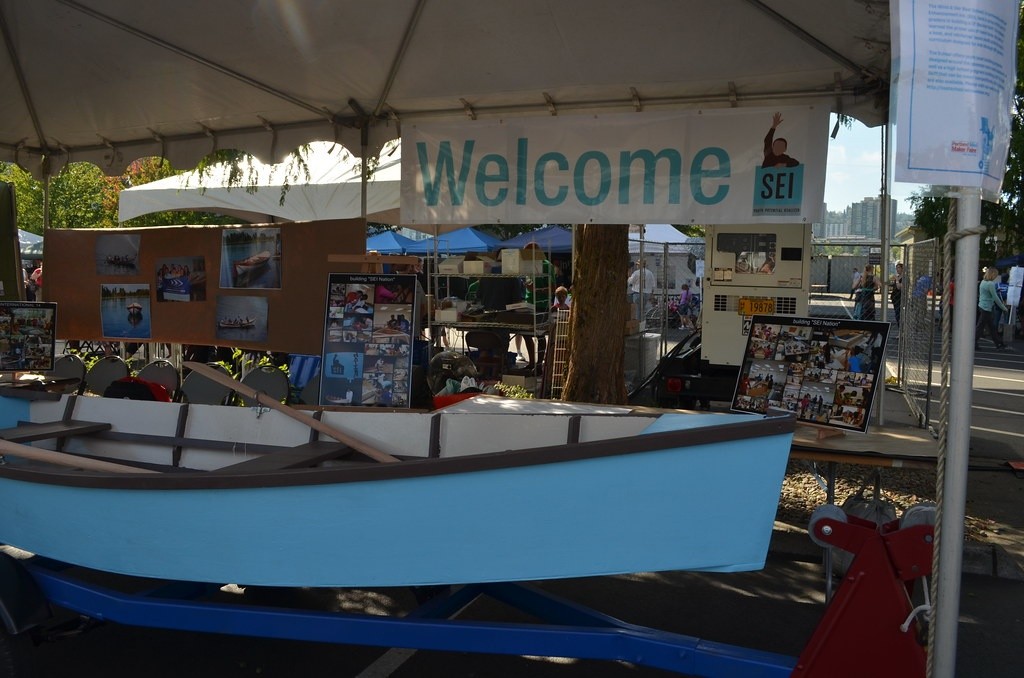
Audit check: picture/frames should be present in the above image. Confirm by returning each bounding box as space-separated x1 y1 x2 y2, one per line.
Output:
730 314 891 434
0 301 57 372
317 272 417 410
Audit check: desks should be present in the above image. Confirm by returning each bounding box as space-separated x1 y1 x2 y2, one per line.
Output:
625 329 649 379
431 395 946 608
0 371 81 392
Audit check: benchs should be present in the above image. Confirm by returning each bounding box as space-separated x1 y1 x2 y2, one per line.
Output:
0 419 111 444
209 441 354 475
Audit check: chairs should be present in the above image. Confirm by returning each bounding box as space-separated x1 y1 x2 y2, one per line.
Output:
135 359 181 403
177 363 233 406
299 374 319 405
233 366 292 406
43 355 87 395
77 355 130 400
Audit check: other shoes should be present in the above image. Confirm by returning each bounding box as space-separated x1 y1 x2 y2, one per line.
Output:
516 353 525 360
997 343 1008 349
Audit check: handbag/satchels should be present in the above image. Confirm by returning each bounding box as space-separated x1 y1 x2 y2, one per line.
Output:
26 279 39 294
677 303 686 315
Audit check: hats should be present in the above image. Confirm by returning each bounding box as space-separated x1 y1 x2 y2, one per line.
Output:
636 258 646 266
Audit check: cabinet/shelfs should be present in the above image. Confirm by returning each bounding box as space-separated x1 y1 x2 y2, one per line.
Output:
426 236 552 399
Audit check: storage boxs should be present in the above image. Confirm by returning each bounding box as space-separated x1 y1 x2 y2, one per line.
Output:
438 255 465 274
435 307 462 322
624 319 639 335
625 304 637 320
497 249 547 274
463 260 492 274
506 303 534 312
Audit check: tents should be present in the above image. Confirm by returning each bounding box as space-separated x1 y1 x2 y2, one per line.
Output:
363 222 689 334
118 139 403 227
17 228 48 259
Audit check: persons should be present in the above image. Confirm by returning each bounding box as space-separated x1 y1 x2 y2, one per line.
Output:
157 264 189 289
106 255 130 263
464 242 569 379
388 253 453 348
627 259 656 321
735 322 878 428
21 261 43 302
677 284 692 331
849 264 881 322
890 264 1010 352
0 309 54 369
330 279 412 405
